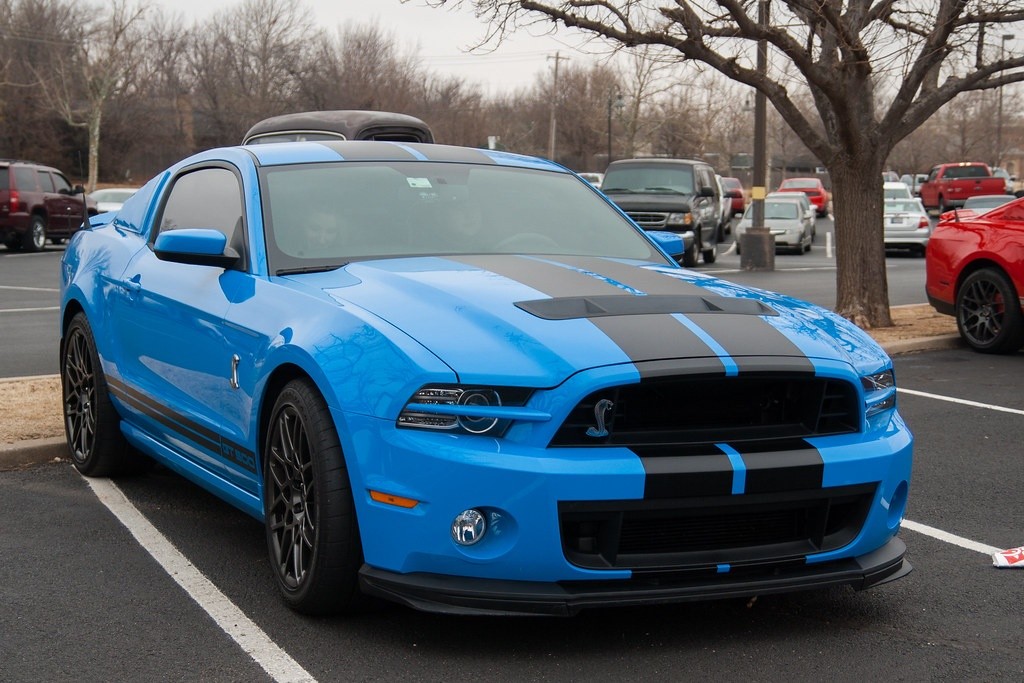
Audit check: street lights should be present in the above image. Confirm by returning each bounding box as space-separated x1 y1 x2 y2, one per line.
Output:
608 84 622 166
997 34 1015 168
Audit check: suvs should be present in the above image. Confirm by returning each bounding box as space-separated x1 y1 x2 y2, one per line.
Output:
0 160 98 252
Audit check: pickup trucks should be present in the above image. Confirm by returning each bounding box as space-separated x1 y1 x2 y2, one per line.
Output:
917 162 1008 218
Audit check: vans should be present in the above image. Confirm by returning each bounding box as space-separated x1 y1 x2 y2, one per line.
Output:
598 160 720 264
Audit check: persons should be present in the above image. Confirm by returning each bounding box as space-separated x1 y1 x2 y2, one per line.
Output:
451 193 483 237
298 199 347 250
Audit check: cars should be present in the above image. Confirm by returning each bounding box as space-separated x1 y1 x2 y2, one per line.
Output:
241 111 436 145
58 142 913 620
576 173 603 192
776 177 830 216
990 167 1017 194
722 178 745 215
766 192 817 235
87 188 141 213
715 174 735 241
884 197 932 252
882 172 923 195
883 182 912 212
733 200 813 253
925 196 1024 353
